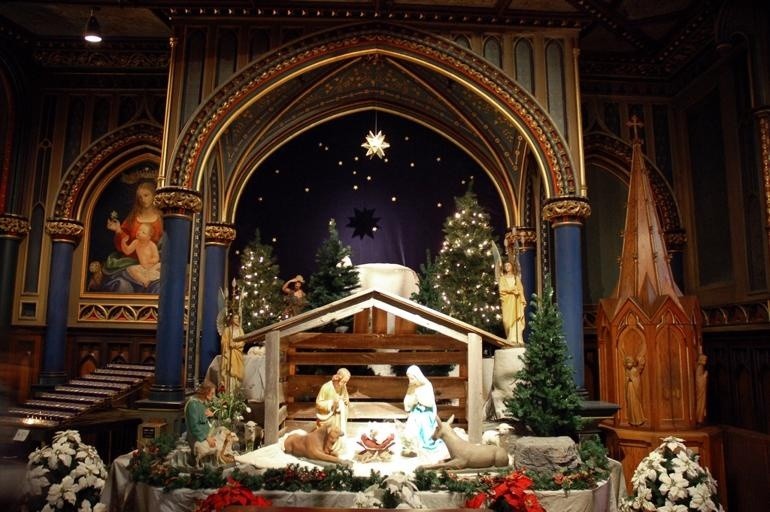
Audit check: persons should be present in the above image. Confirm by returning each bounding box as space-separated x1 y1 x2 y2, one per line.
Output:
184 378 216 449
315 368 351 455
121 223 159 288
404 365 442 450
624 357 648 426
103 183 163 291
695 354 708 423
283 275 307 298
498 262 527 345
220 313 245 391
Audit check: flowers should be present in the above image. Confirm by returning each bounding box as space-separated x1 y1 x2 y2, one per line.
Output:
21 429 108 511
125 432 611 511
619 436 726 512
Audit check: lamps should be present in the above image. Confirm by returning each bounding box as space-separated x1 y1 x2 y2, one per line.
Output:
82 1 102 44
360 110 391 161
